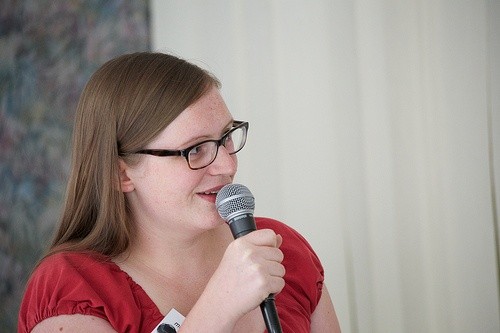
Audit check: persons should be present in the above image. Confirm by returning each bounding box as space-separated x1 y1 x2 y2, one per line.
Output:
15 51 341 333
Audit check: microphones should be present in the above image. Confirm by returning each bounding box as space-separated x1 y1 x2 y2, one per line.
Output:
216 183 286 333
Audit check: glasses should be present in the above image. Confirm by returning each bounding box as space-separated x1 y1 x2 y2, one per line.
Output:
123 119 250 171
